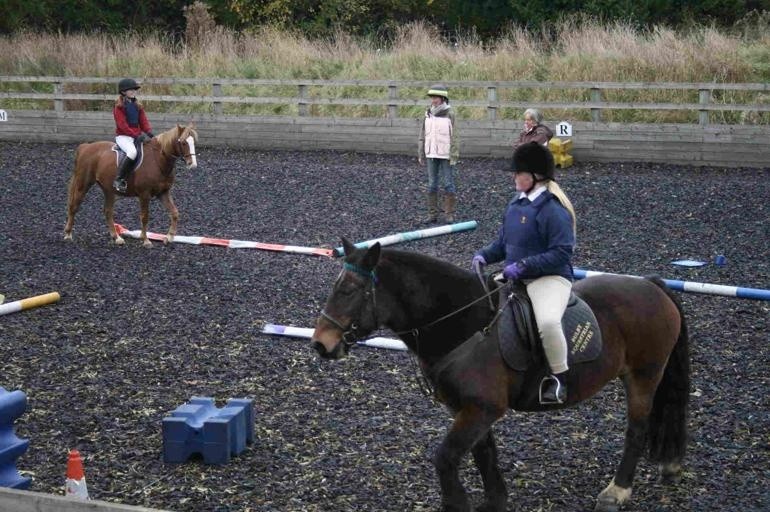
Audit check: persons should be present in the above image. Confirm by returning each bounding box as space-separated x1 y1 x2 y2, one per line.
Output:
473 140 576 402
521 107 554 146
418 83 460 222
113 79 155 193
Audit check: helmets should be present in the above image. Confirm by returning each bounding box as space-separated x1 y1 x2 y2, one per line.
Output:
119 79 141 93
426 84 448 100
502 140 555 181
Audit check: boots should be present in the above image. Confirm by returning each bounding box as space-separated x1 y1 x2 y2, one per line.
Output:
112 156 135 193
444 193 457 223
423 192 439 223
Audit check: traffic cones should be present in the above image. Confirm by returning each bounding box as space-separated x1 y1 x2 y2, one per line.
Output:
61 449 92 502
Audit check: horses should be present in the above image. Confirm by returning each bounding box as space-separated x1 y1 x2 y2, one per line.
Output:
62 120 199 250
310 235 690 512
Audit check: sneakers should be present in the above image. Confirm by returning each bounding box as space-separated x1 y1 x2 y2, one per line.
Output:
543 370 568 401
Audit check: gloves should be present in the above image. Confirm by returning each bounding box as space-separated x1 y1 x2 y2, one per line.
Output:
503 261 527 281
137 132 155 144
471 255 487 274
